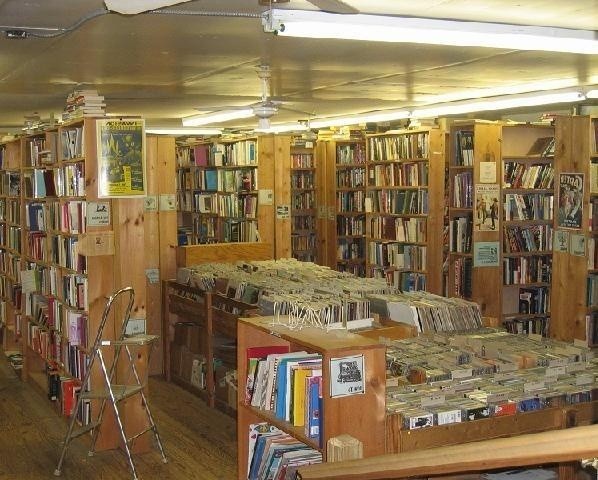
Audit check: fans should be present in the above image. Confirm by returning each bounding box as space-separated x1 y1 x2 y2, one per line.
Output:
182 64 330 117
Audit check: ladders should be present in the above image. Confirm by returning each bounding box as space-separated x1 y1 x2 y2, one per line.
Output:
53 286 168 480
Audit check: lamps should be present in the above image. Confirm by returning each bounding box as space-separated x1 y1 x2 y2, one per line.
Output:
260 1 598 56
182 106 254 127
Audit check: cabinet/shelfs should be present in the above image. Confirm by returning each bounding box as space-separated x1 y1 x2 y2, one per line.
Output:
2 115 151 455
160 242 497 421
234 315 598 480
146 112 596 376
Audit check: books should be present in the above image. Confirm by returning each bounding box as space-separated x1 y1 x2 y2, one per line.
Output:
501 132 598 346
368 291 597 427
289 134 317 263
447 130 471 299
174 139 257 244
65 89 106 118
0 127 91 427
174 255 400 323
245 345 322 480
179 350 238 408
366 136 426 290
336 144 366 277
